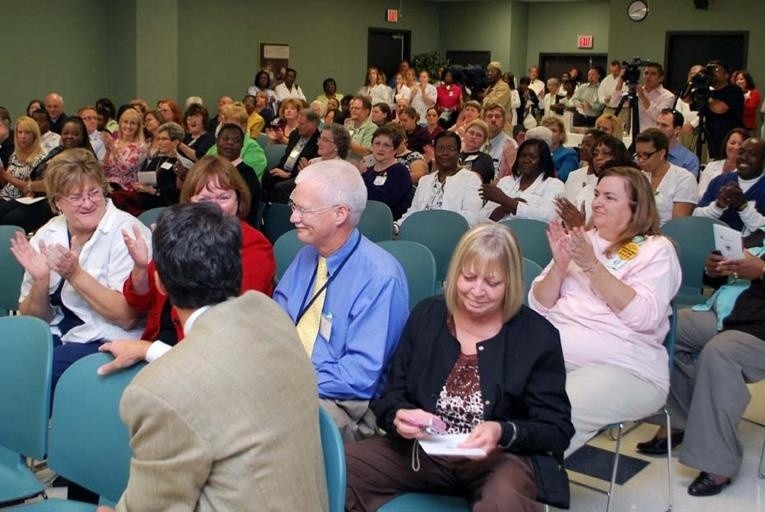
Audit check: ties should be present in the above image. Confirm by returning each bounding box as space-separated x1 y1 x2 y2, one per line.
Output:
296 254 328 360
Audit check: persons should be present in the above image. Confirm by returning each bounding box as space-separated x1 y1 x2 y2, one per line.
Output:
1 55 765 511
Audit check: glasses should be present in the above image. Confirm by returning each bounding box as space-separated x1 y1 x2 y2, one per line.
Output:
60 187 104 207
632 148 660 160
287 200 337 218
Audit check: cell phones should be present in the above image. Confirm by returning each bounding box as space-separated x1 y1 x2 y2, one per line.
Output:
711 250 723 261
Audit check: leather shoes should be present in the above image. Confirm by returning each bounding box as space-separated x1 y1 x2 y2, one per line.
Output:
637 431 684 453
688 471 731 496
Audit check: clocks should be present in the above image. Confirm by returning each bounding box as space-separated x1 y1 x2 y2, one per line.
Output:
627 0 650 22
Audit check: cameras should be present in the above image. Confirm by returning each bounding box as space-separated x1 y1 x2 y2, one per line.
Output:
406 414 447 435
621 56 650 84
679 63 720 102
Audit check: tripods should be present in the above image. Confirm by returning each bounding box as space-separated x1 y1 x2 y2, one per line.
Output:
689 102 721 183
613 84 640 152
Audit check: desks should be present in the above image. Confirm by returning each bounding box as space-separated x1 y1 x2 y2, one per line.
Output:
554 130 633 153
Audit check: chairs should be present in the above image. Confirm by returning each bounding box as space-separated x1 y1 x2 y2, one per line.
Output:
0 314 59 508
0 223 30 317
11 340 158 512
131 206 170 233
251 127 364 179
